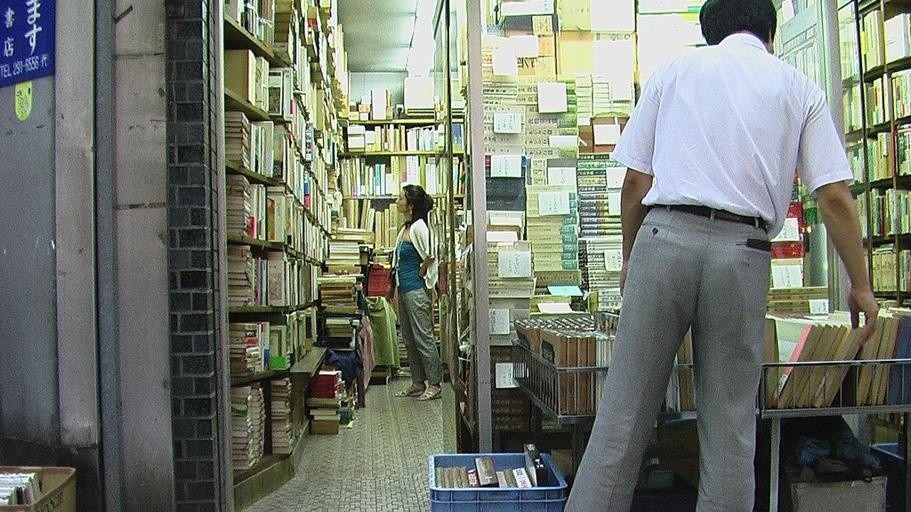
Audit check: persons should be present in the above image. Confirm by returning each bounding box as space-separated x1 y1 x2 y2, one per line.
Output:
385 185 443 401
561 1 880 510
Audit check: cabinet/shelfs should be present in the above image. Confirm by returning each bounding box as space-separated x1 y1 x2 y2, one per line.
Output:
224 1 911 512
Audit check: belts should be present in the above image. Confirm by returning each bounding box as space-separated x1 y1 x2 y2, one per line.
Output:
651 202 767 229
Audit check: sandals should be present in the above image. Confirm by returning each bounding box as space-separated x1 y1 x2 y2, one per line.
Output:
393 382 427 396
416 383 443 402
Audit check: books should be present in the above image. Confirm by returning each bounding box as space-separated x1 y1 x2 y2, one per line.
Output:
224 1 911 491
0 468 44 505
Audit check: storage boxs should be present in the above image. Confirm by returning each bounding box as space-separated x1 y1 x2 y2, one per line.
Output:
1 466 76 512
